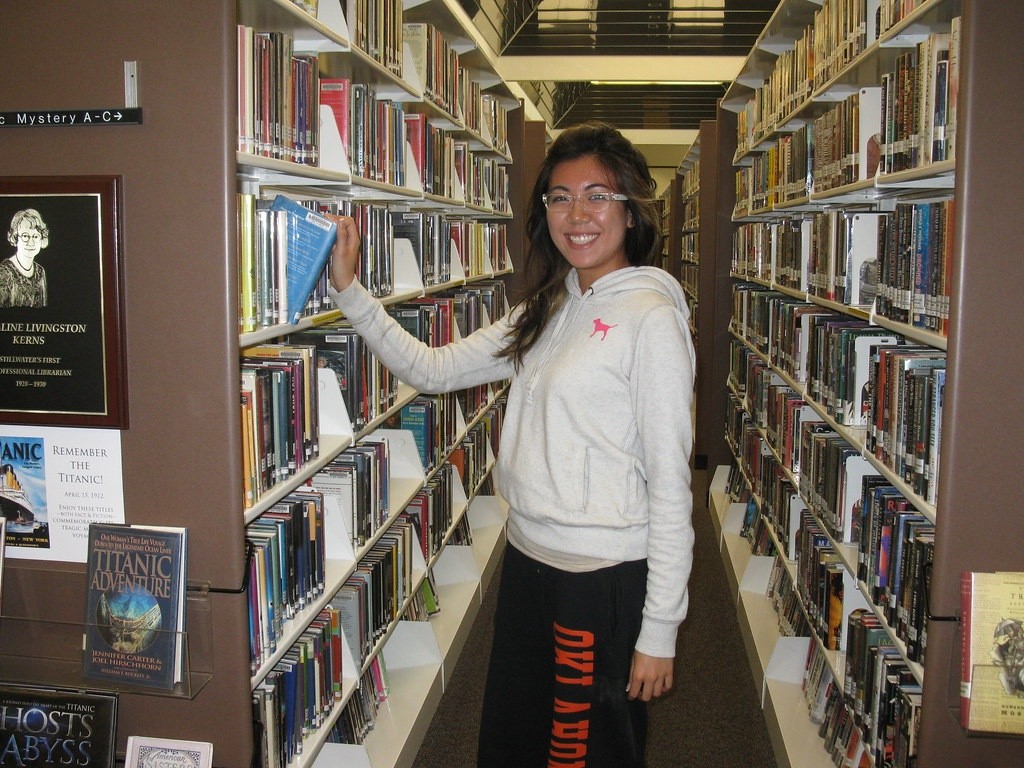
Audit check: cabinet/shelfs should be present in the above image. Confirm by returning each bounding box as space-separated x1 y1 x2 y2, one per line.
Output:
0 2 556 767
649 1 1024 768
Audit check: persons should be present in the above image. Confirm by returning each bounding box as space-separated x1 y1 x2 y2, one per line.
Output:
320 123 697 768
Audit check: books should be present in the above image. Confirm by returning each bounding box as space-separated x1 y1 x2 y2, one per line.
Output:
236 0 1023 767
83 522 189 690
0 681 120 768
125 736 214 768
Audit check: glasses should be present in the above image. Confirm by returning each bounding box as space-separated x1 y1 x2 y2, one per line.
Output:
541 192 629 213
